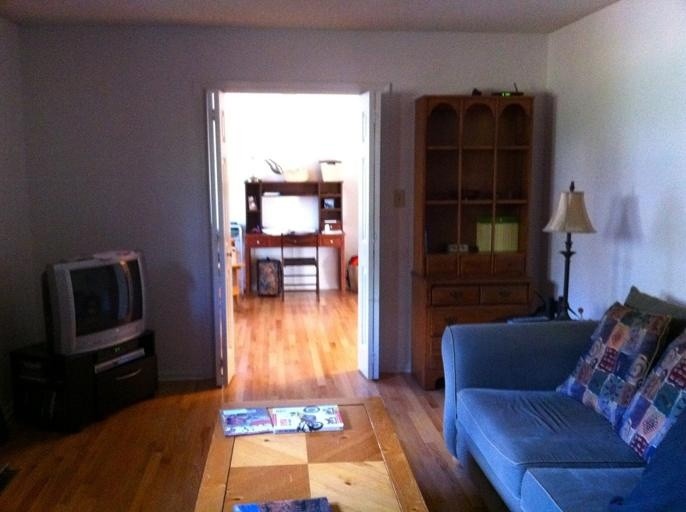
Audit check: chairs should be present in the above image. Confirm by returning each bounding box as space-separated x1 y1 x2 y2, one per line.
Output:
279 233 319 301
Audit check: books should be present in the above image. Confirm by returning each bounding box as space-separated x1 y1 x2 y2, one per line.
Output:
219 406 274 437
266 402 345 433
234 497 330 511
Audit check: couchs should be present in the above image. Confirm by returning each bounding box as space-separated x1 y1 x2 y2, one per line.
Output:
440 287 686 511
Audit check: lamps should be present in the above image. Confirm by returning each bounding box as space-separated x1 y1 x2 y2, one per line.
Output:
541 180 598 321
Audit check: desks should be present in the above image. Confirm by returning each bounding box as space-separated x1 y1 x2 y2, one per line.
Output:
244 231 346 297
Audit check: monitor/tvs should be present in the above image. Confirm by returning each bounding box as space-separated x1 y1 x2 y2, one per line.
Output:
41 251 145 357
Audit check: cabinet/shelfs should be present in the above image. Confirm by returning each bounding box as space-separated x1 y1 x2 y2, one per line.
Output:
7 327 158 432
247 179 343 233
413 93 536 278
410 271 533 390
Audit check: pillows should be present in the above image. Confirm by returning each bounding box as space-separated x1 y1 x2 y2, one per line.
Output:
555 300 686 465
